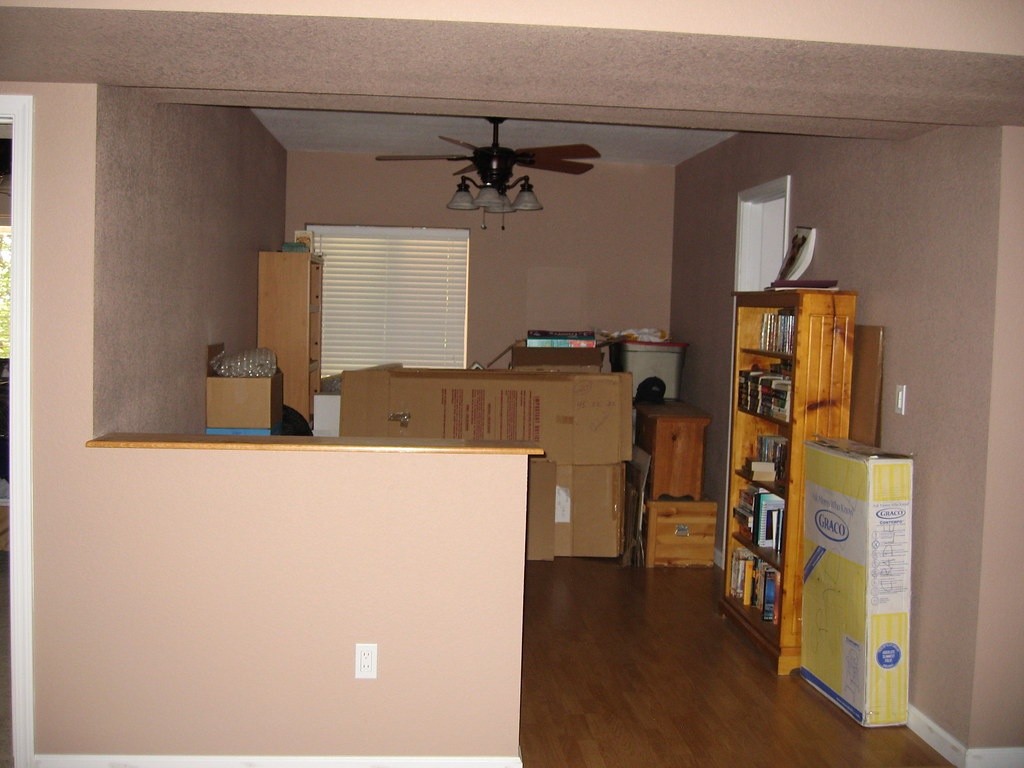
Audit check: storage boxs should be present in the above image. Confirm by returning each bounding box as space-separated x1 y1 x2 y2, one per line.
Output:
803 433 914 728
206 343 284 436
312 331 721 568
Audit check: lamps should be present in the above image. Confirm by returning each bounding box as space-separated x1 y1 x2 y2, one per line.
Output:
447 178 543 231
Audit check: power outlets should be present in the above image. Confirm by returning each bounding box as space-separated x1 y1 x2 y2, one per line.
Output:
353 644 378 678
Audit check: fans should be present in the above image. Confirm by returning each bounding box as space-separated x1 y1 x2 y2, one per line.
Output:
375 117 601 230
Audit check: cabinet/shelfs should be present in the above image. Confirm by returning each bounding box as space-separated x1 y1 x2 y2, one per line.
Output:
718 286 860 677
635 403 714 501
256 251 323 431
